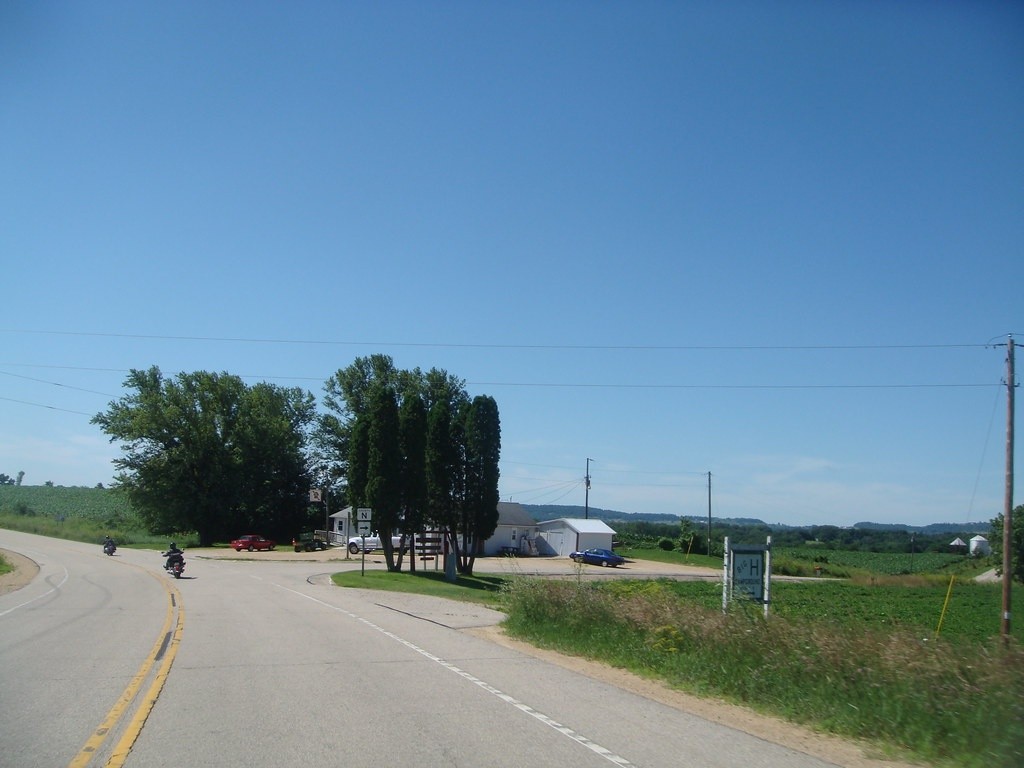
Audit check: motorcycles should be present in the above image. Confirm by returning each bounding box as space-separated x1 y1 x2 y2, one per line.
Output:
103 539 116 556
161 548 186 579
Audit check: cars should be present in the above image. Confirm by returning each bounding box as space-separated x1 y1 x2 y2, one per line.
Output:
231 535 275 552
569 548 625 567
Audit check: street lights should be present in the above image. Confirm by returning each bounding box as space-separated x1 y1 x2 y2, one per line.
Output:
586 458 594 518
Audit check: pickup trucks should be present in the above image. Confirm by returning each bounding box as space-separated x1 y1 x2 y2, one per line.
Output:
349 530 411 555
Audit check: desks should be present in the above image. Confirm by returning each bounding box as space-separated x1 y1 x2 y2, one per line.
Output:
501 547 520 557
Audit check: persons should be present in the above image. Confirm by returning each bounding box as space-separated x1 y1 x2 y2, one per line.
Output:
163 542 185 569
102 535 114 550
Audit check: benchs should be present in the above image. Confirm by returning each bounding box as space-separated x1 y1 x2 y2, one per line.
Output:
497 551 518 552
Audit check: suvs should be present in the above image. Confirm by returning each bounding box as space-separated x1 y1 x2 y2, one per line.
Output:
292 532 328 552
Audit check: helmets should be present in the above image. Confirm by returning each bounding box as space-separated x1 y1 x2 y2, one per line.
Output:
105 535 109 539
170 542 176 549
